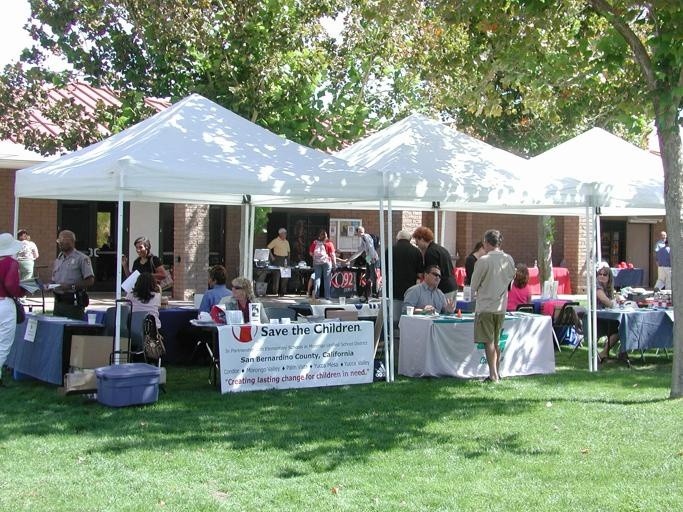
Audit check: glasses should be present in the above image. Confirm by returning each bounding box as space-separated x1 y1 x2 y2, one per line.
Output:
232 285 242 289
431 271 441 277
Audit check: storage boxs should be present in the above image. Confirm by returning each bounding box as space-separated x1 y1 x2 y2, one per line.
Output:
90 362 162 409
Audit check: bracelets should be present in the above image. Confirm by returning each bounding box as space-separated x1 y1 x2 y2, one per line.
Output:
71 284 75 291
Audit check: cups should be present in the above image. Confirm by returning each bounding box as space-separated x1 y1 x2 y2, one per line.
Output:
87 313 95 324
269 317 278 324
339 296 344 305
161 294 168 309
197 311 211 320
406 305 413 317
280 317 290 324
542 280 559 299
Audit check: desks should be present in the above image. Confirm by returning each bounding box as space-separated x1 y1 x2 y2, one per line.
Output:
5 261 675 396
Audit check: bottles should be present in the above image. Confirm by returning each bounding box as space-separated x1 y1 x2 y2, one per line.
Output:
283 258 287 267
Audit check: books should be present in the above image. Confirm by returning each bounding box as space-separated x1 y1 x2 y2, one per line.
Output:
20 277 43 294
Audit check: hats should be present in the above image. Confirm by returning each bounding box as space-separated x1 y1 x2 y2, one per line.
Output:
0 233 22 257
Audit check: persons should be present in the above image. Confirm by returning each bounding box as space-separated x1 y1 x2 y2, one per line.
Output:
44 229 95 319
380 230 423 337
267 228 291 296
130 237 168 280
401 264 456 314
123 271 162 330
582 267 632 367
466 241 486 282
218 276 269 324
654 240 671 290
413 228 457 302
305 231 348 299
653 230 667 253
470 230 515 382
507 263 530 310
0 232 28 392
346 227 379 295
196 265 232 318
17 229 38 281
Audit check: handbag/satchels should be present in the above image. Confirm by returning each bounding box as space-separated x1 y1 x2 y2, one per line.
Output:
56 289 89 307
14 299 25 324
363 233 380 250
143 315 166 359
150 256 173 290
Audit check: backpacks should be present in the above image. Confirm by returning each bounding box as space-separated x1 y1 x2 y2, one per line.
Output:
313 240 328 265
